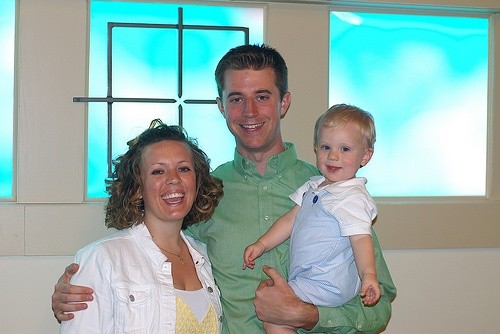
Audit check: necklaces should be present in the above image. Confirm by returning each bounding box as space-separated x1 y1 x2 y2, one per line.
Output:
156 241 185 265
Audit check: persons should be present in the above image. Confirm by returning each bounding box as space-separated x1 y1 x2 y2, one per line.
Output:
51 44 396 334
242 103 382 334
59 118 224 334
